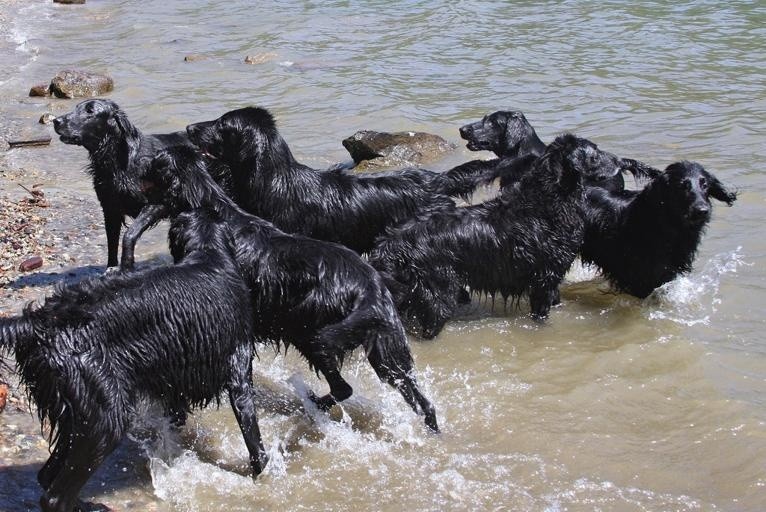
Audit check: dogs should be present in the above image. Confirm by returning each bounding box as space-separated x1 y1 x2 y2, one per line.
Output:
0 207 269 512
53 99 235 271
135 142 439 434
580 160 742 299
361 133 626 340
459 111 662 192
186 107 501 305
